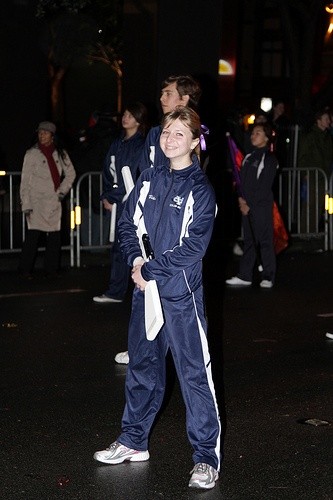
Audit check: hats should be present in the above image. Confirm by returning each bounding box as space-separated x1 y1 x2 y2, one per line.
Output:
36 122 56 132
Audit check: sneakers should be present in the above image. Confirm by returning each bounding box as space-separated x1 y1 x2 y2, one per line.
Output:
94 441 150 464
115 351 130 364
260 280 273 288
189 463 219 489
93 294 122 303
326 329 333 338
226 277 251 286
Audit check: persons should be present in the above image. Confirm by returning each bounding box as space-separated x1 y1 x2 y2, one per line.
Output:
92 104 222 489
115 75 208 365
225 123 278 288
234 98 333 254
93 105 145 303
17 121 76 282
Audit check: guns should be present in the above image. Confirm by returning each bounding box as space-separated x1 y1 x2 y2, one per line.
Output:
121 166 164 341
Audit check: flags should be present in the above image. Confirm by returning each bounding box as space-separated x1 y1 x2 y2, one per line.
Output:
226 132 288 254
